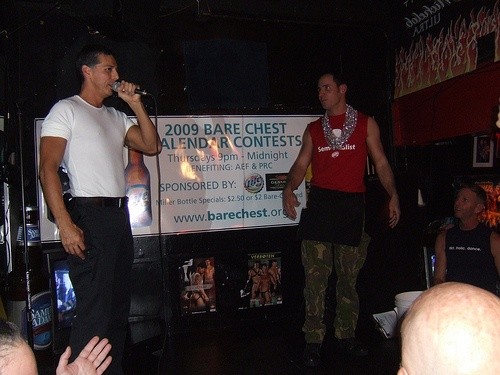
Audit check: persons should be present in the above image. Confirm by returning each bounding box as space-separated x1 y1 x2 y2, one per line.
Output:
245 259 282 305
182 259 215 309
283 71 400 367
397 281 500 375
0 320 113 375
434 185 500 296
39 48 161 375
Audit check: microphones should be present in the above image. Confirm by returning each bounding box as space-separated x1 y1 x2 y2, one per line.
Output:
112 82 153 97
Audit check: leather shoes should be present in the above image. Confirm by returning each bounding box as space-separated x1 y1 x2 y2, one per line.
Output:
303 343 320 367
336 337 368 355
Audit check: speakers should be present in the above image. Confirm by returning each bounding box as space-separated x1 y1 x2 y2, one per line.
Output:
130 253 163 343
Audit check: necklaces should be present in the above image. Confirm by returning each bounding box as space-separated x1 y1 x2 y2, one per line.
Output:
323 103 357 149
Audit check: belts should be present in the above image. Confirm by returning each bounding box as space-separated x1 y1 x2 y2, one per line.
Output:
68 197 127 208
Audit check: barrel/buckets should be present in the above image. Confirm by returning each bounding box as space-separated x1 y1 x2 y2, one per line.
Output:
395 291 423 329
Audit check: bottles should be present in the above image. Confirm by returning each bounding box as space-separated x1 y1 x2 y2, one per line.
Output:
13 202 56 366
124 145 153 227
